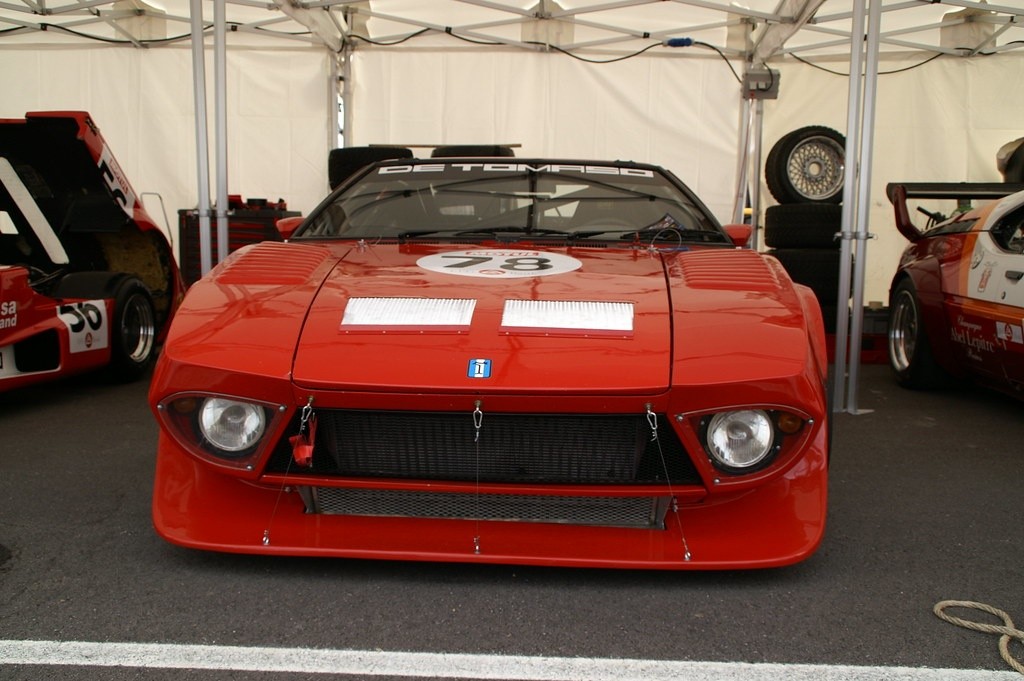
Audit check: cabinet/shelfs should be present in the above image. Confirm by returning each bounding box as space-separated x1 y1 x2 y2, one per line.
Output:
177 210 301 288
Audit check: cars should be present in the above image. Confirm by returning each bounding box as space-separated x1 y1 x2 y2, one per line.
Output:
886 136 1024 390
0 109 186 405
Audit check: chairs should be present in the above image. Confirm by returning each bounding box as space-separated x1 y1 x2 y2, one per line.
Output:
565 181 653 232
347 180 439 229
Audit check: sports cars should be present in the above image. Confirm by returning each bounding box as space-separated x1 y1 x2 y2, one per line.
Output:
150 142 831 574
